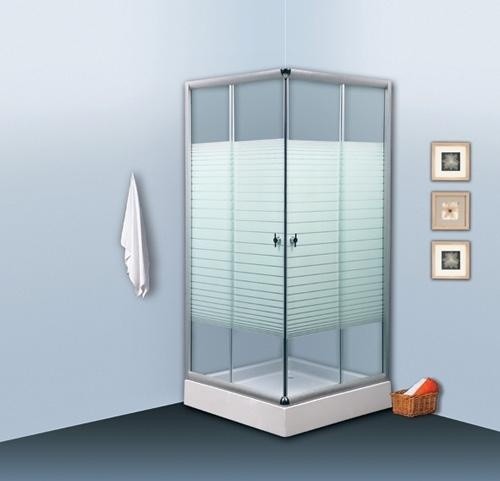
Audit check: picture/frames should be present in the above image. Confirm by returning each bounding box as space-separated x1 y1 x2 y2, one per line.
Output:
431 141 473 281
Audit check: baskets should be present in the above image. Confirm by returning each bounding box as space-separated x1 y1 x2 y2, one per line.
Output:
388 386 439 418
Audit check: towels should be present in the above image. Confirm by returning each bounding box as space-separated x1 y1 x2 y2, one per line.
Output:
121 170 152 299
